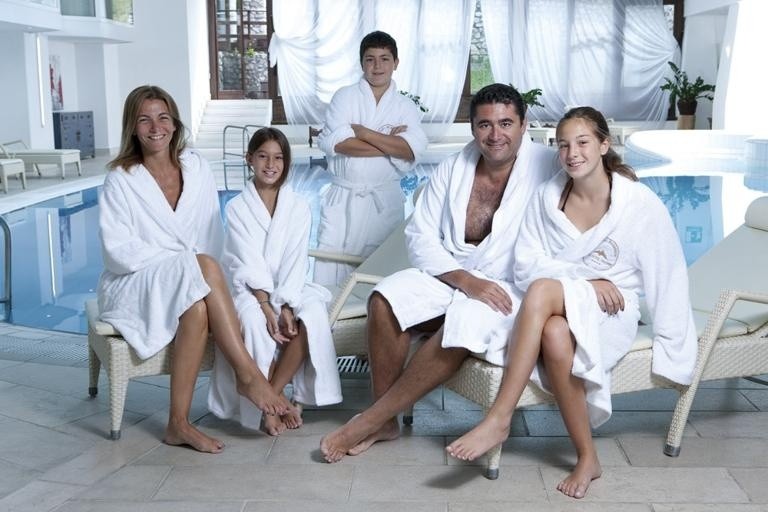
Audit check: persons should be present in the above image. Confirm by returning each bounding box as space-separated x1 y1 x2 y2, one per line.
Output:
445 106 700 500
320 83 567 462
208 126 343 436
315 31 427 285
97 82 289 453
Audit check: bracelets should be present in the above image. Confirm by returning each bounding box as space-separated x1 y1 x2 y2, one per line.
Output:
259 301 270 304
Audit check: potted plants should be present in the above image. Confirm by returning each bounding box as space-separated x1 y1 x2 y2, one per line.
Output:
659 60 715 115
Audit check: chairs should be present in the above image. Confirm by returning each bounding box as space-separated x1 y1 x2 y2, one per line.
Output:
1 139 82 195
86 182 427 438
398 193 768 479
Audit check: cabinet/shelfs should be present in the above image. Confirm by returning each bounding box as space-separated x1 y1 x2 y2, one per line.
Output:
52 111 95 160
